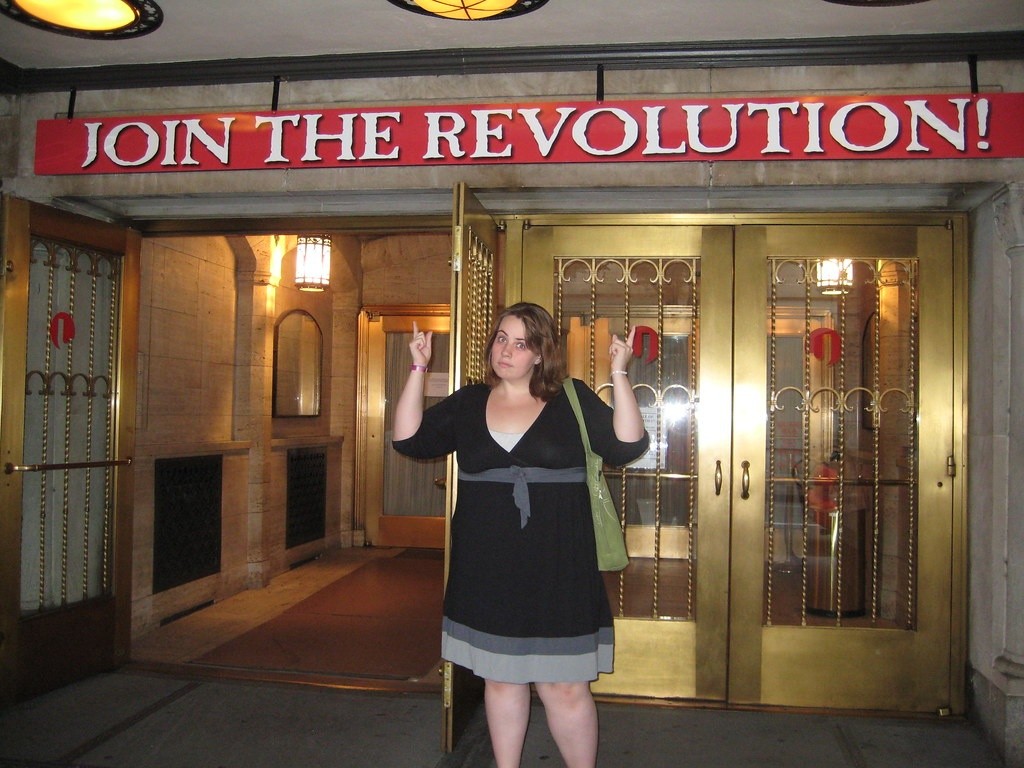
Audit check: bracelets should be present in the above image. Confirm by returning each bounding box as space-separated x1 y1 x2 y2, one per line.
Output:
408 363 427 375
609 370 629 377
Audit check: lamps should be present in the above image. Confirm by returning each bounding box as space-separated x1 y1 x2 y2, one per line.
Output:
387 0 550 21
0 0 164 40
816 257 855 297
294 234 333 293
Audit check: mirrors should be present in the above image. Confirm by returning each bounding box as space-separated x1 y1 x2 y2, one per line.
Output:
271 306 324 418
861 311 876 432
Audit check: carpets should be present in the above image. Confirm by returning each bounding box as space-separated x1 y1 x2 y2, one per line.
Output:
189 548 445 679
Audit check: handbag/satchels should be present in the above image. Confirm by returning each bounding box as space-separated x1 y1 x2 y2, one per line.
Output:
560 377 630 572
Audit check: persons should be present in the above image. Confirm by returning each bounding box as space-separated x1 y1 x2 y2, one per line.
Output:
393 303 648 768
